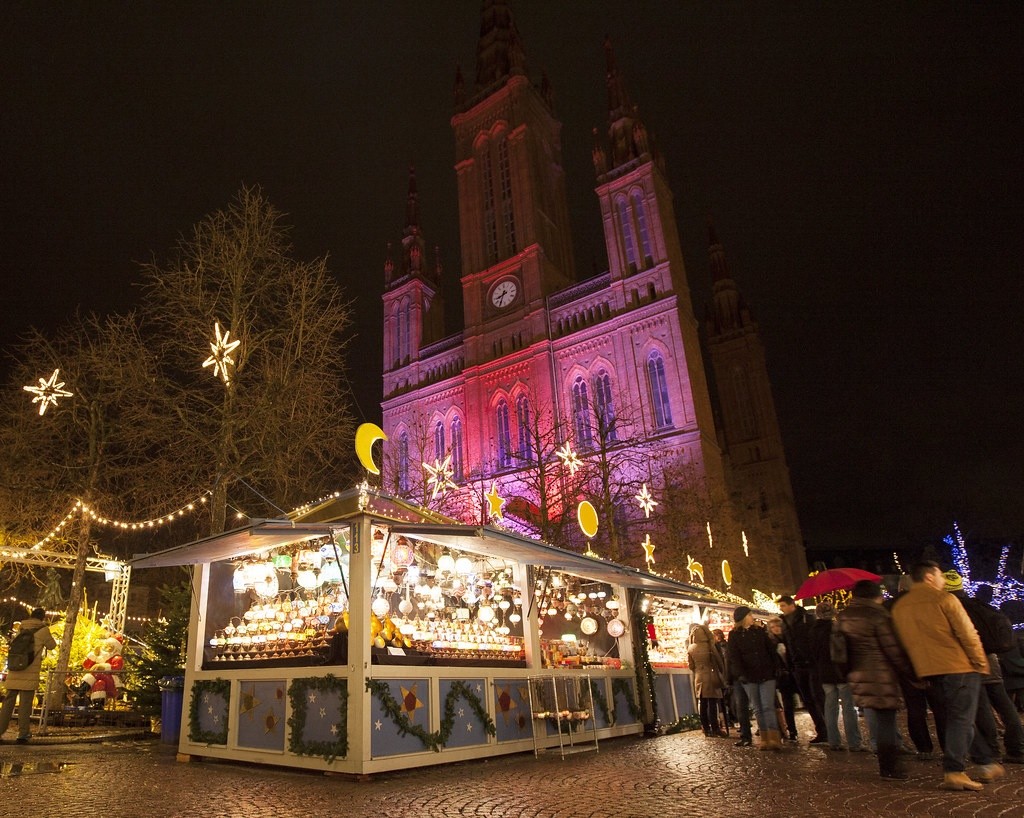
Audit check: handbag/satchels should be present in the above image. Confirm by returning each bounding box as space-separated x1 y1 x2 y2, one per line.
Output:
710 665 722 689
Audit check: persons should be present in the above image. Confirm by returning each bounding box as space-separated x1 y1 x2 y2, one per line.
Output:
810 603 867 751
768 616 801 742
974 585 1024 714
884 574 943 760
688 625 727 739
942 570 1024 764
727 607 781 751
712 630 741 729
70 636 124 710
830 580 917 781
892 560 1005 787
0 608 55 737
777 596 827 744
685 623 705 733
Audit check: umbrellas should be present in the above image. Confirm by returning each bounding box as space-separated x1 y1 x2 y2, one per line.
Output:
794 562 883 606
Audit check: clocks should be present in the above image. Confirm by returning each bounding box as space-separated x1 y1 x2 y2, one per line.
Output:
491 281 516 308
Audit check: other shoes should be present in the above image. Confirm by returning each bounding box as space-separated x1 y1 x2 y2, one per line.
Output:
711 725 726 738
827 745 848 751
701 726 712 738
881 767 909 781
16 733 32 740
1001 752 1024 764
977 762 1006 783
735 737 752 747
727 721 735 728
848 744 869 752
915 749 933 761
788 731 798 742
943 770 984 791
986 748 1003 763
809 735 829 744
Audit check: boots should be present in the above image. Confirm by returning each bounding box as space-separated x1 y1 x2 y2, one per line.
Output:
776 708 790 745
758 727 782 751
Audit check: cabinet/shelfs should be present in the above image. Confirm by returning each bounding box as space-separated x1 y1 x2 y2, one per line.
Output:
528 672 599 760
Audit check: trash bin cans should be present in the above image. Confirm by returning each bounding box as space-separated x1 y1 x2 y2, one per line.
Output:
159 686 184 745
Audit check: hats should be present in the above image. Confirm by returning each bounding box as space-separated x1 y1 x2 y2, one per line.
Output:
852 580 881 599
734 606 752 623
942 569 963 592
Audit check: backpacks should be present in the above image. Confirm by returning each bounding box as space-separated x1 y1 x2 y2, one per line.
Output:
7 625 47 672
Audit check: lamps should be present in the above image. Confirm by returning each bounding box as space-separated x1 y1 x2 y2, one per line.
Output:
210 534 619 661
